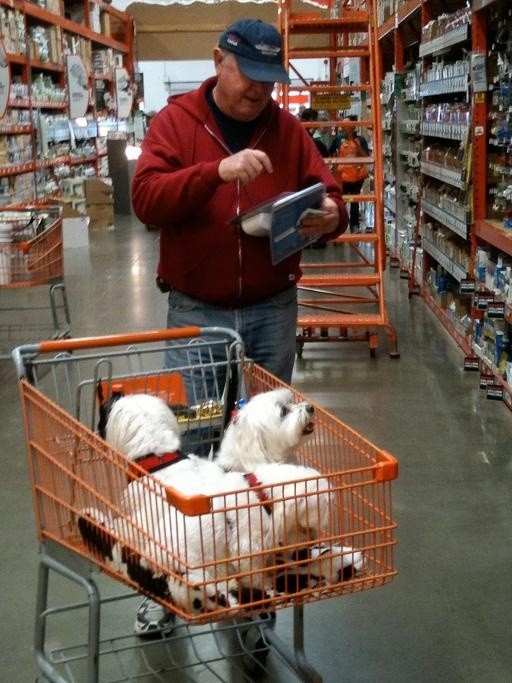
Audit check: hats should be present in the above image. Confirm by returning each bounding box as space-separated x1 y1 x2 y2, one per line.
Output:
219 19 292 85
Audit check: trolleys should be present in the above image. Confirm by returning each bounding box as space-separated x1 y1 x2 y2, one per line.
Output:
0 201 78 387
2 321 409 682
275 0 405 366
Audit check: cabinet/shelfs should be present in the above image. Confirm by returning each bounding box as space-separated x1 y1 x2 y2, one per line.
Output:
361 0 511 412
0 1 135 219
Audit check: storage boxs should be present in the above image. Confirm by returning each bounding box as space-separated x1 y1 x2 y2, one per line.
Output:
87 204 115 230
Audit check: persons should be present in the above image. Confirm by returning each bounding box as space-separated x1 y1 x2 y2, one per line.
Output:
293 102 371 250
130 19 350 671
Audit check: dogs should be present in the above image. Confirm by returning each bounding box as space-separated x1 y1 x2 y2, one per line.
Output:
75 386 370 625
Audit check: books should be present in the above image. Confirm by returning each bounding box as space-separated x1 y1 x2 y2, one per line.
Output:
268 182 325 267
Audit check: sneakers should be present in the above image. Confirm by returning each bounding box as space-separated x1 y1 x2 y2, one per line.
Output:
133 596 173 638
